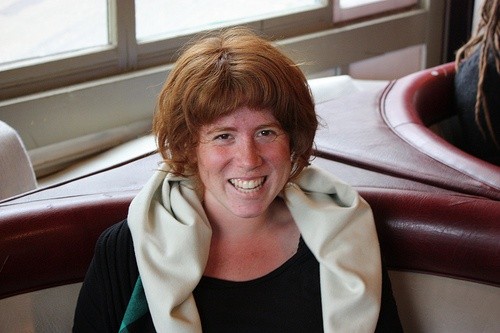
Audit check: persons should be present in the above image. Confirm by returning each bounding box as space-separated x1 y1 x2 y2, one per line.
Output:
446 0 500 167
70 24 407 333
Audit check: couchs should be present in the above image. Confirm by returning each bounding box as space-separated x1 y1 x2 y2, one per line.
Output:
0 53 500 332
